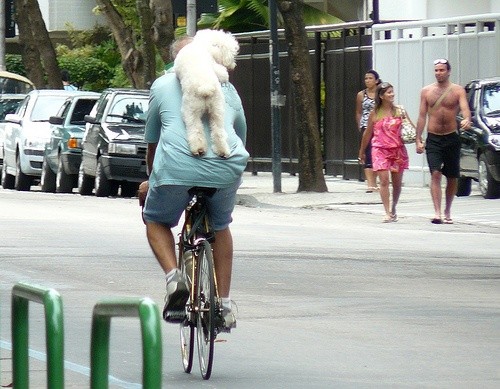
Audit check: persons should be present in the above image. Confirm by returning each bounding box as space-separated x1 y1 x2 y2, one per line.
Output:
416 59 471 224
138 36 249 329
359 82 423 223
356 70 382 193
60 70 78 91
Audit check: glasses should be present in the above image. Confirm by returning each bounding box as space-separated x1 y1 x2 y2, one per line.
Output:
433 58 449 65
381 82 392 88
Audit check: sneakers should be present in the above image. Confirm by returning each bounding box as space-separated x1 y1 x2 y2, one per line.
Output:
163 272 190 324
216 299 238 333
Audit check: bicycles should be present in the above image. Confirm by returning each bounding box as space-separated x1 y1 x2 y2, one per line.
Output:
143 185 223 379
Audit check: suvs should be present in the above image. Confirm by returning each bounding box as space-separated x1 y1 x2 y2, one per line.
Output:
453 77 500 199
78 88 153 197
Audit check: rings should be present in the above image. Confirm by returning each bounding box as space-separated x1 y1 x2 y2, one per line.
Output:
358 158 361 161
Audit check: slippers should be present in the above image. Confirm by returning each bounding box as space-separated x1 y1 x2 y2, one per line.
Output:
367 186 373 193
443 218 453 224
391 212 398 222
431 217 442 224
382 216 390 223
373 186 380 192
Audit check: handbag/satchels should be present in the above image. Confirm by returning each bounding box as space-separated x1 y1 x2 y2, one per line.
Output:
401 117 417 144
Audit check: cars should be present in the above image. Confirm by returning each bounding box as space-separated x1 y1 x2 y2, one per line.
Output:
0 94 30 185
2 90 97 191
41 94 101 193
